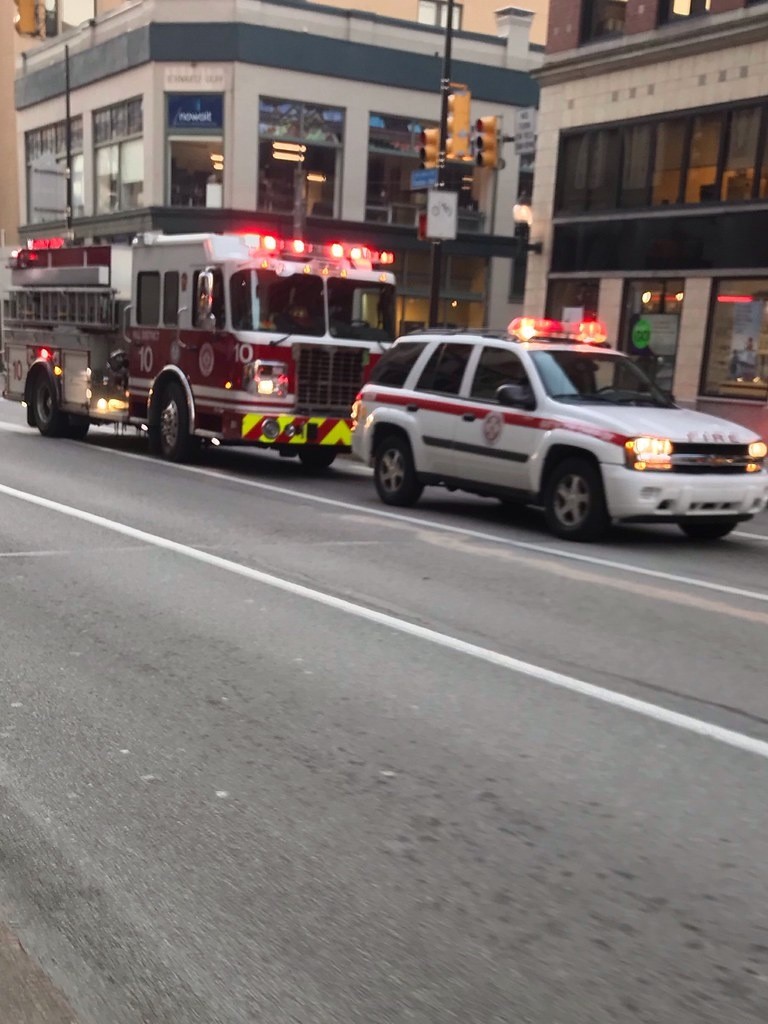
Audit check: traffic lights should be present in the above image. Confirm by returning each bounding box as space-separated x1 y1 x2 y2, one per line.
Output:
419 126 440 172
446 91 470 162
475 115 500 170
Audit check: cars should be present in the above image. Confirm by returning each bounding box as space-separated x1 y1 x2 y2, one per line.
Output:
344 316 768 548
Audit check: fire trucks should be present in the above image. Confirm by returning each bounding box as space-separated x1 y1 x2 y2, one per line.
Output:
1 202 400 467
